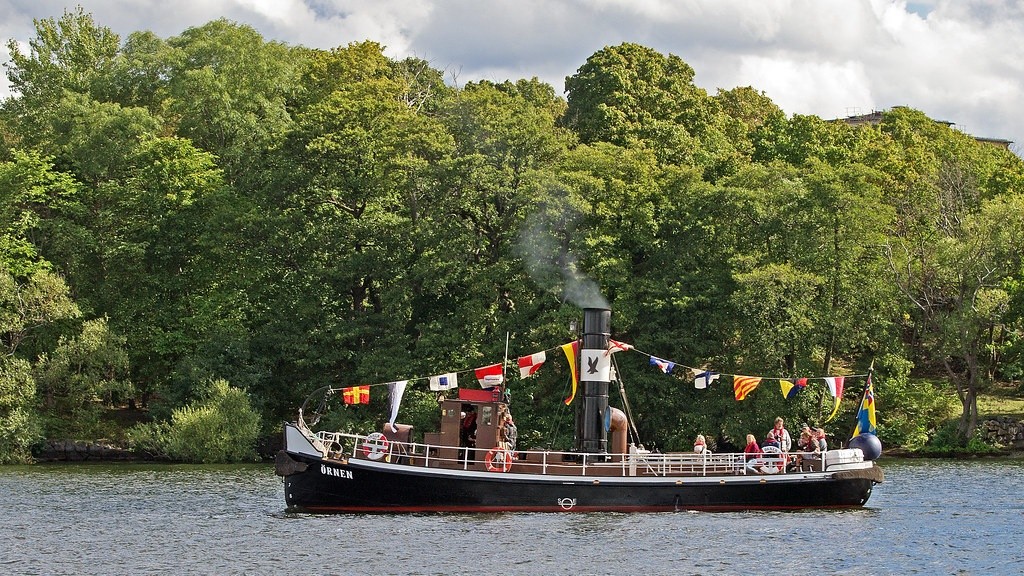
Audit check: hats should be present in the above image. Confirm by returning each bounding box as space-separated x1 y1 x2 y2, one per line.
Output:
464 406 474 413
810 426 818 432
800 423 809 430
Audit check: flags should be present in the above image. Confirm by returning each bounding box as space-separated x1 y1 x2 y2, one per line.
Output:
849 378 877 442
343 338 844 434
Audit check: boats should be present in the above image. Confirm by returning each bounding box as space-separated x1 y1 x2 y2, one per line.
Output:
274 308 885 514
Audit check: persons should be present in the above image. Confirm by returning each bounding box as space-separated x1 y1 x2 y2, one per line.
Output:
460 407 477 458
761 417 792 474
735 434 762 474
797 423 827 472
694 435 707 462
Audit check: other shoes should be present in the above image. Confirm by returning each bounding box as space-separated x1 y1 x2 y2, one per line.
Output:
789 467 800 472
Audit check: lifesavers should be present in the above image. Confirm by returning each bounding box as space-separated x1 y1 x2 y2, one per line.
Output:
361 431 390 462
483 446 514 473
754 445 787 477
